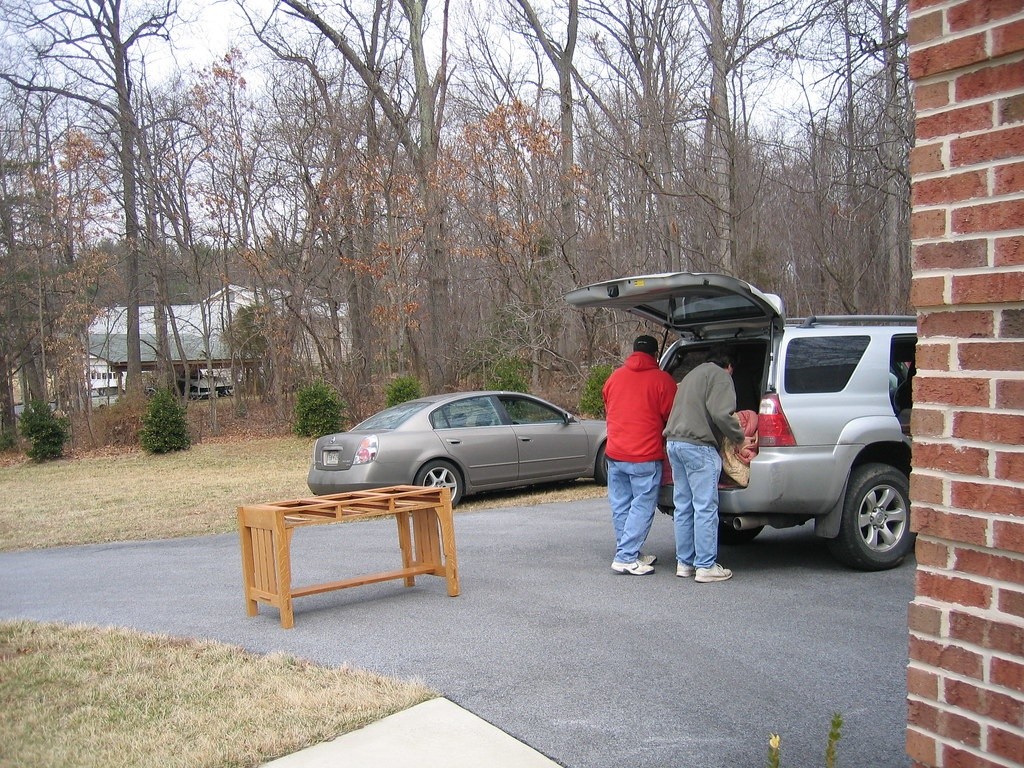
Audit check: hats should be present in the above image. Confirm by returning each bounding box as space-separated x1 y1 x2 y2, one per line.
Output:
633 335 660 353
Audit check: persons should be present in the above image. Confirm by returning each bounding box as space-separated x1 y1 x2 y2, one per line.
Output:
602 335 678 575
661 351 756 582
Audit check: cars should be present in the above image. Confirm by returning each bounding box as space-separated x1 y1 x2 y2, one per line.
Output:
306 391 607 508
87 385 127 410
173 366 233 400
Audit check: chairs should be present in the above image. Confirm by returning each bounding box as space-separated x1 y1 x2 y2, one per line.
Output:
452 413 467 426
475 412 492 426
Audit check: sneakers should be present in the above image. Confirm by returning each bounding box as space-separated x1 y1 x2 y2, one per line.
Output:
637 553 657 565
610 558 655 575
676 560 696 577
695 562 732 583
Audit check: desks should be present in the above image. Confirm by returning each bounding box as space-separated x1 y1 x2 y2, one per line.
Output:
238 485 461 626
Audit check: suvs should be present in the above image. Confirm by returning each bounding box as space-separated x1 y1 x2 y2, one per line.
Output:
563 273 917 572
